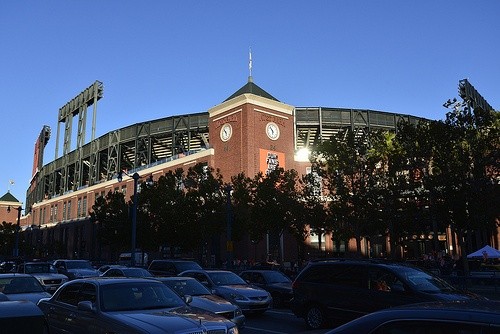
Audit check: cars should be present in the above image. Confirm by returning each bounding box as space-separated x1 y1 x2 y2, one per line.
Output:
0 264 152 334
36 277 240 334
239 271 295 309
179 269 273 315
325 299 500 334
155 276 247 329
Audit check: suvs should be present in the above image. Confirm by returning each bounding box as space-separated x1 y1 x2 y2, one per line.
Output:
54 259 102 279
147 259 202 277
288 258 488 329
19 261 69 292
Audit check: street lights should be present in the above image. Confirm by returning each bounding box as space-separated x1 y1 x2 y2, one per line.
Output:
118 168 155 267
6 204 33 273
